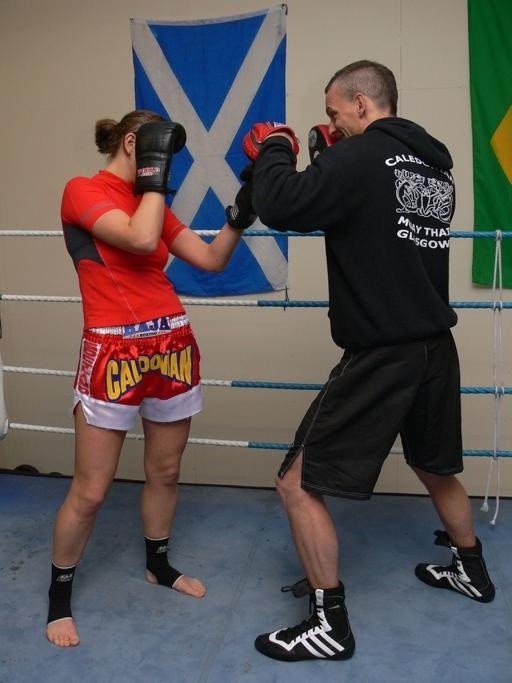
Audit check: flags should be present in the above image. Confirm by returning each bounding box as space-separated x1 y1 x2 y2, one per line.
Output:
468 0 512 289
130 5 289 296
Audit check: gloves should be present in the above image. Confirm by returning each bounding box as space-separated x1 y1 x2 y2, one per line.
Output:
134 122 187 193
308 124 335 162
225 121 299 231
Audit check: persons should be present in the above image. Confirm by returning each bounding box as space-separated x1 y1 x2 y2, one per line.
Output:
46 111 257 646
242 61 495 662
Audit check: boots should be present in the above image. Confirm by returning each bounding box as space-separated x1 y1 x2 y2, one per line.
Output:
254 579 356 663
414 530 496 603
